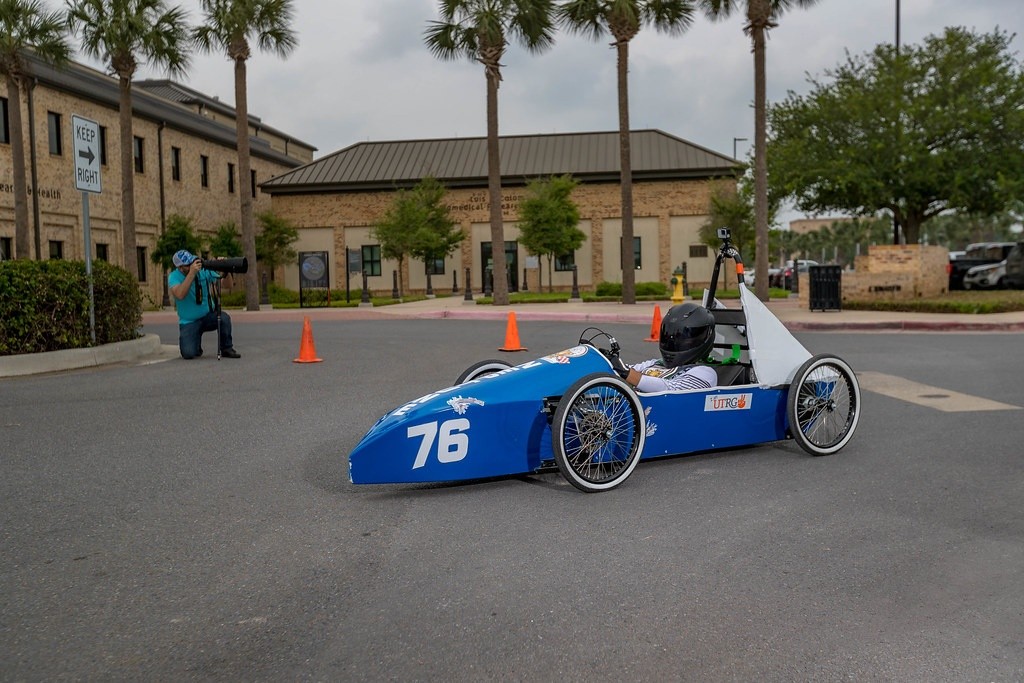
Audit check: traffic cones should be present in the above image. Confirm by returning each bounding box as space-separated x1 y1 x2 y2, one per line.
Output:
292 314 324 363
643 304 663 342
497 311 527 352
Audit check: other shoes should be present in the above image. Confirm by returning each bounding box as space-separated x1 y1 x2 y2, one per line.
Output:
221 347 241 358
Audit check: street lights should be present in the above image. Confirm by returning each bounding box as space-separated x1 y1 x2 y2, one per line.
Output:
733 138 748 159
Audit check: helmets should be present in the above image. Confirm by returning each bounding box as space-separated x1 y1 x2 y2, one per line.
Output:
660 303 715 368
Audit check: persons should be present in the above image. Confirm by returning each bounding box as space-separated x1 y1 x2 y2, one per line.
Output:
169 250 241 359
579 303 718 394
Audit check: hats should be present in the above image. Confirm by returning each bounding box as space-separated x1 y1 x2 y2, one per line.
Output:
173 250 199 267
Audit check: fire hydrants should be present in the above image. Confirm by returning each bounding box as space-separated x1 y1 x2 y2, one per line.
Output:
670 265 687 305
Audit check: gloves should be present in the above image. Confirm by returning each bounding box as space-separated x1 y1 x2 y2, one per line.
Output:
600 347 629 378
580 338 596 348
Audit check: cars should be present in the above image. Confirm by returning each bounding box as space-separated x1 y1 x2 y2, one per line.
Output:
743 259 818 293
948 242 1024 291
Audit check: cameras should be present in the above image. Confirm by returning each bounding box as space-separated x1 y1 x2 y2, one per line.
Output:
194 257 249 273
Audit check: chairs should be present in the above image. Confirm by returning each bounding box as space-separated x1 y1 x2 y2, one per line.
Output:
698 361 747 388
